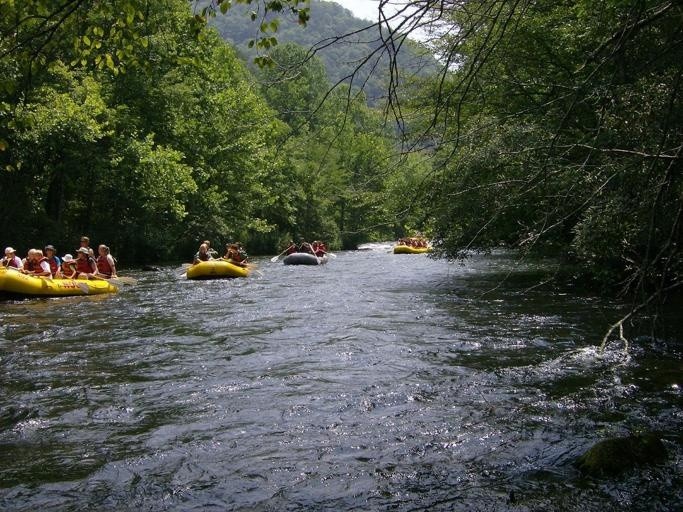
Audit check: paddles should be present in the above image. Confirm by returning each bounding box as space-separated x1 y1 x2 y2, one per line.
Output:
319 248 337 260
8 265 54 284
310 245 322 266
271 244 295 262
176 264 193 275
112 276 138 286
92 274 124 288
61 272 90 294
222 258 258 269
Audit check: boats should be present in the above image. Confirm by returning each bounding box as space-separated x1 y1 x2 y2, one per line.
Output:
282 252 331 267
393 244 433 254
185 260 249 281
0 264 122 298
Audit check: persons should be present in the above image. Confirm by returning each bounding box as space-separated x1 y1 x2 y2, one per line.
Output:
193 240 218 265
286 240 326 257
0 237 116 279
399 237 427 248
224 242 248 266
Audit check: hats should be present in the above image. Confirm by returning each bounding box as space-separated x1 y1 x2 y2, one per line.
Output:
62 254 74 262
45 245 55 254
76 247 90 255
5 246 16 254
